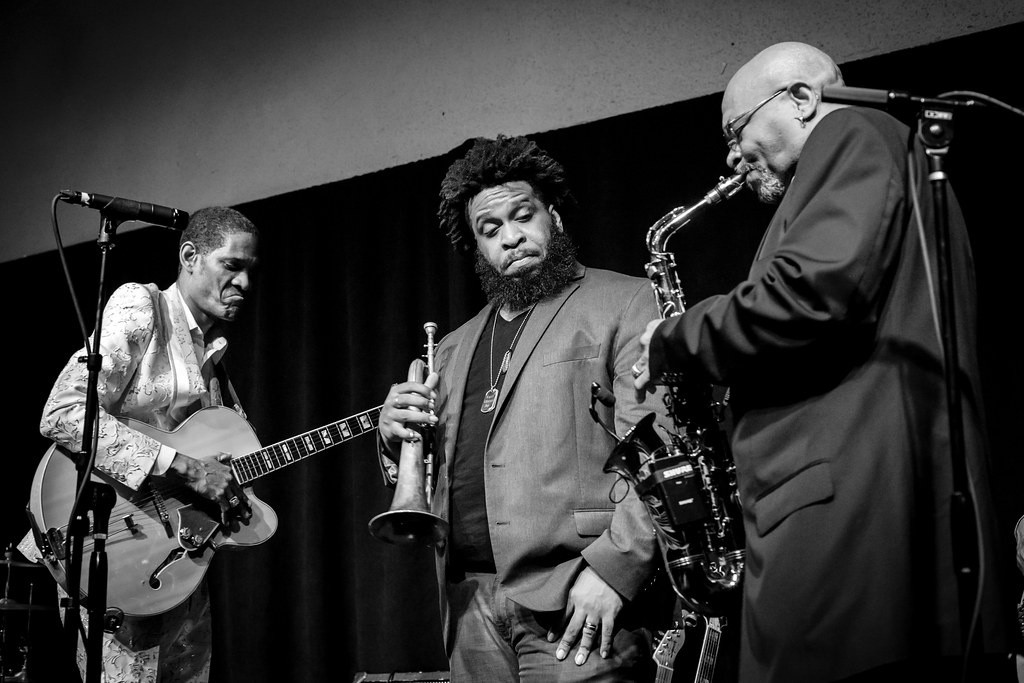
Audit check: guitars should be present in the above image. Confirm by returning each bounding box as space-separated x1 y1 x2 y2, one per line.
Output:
24 404 384 623
692 616 722 682
645 626 685 683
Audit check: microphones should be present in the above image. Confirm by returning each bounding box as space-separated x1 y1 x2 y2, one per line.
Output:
62 190 190 230
592 382 616 408
822 85 910 105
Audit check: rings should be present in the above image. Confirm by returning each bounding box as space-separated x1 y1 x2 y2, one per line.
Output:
631 363 641 376
584 622 596 631
395 396 402 408
229 495 240 507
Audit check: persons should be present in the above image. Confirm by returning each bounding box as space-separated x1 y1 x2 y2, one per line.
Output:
39 206 258 683
376 130 681 682
629 38 1007 682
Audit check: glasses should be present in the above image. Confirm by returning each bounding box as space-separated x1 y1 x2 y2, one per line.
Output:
723 85 820 143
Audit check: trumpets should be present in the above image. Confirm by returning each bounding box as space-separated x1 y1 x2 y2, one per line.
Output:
367 323 450 548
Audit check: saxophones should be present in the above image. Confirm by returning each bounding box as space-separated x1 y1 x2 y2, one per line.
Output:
601 176 752 615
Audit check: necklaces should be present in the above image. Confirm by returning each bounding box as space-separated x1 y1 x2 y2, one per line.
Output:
480 298 538 413
